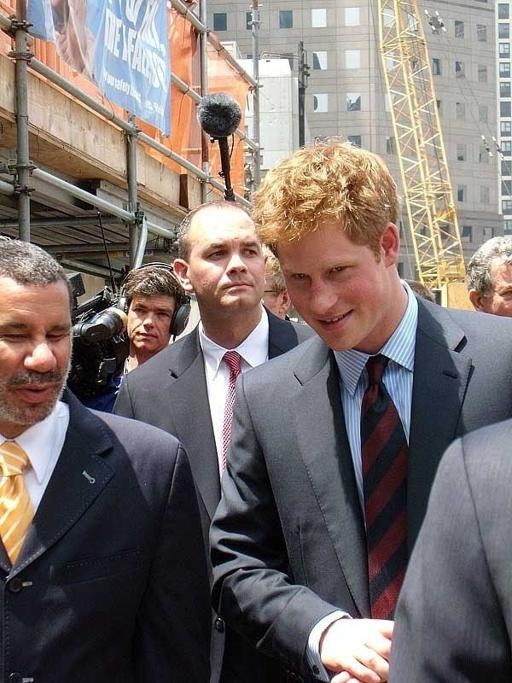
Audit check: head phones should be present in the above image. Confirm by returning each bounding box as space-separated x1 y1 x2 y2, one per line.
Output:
116 261 191 335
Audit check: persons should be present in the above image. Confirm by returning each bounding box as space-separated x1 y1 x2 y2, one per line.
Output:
210 136 511 683
1 235 213 683
397 275 438 303
255 245 293 321
76 267 187 417
388 414 512 682
463 240 511 320
110 202 323 683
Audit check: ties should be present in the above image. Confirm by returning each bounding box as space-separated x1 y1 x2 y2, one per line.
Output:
221 350 243 473
360 353 408 620
0 441 34 567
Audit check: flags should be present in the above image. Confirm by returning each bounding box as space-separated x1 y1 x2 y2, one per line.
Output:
25 2 173 138
